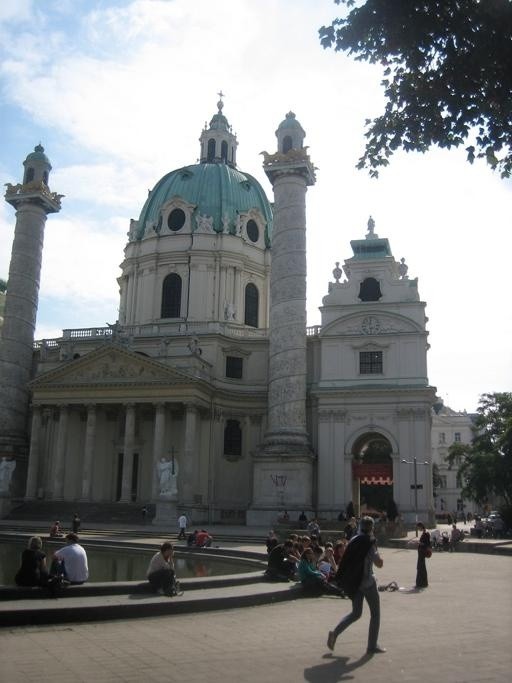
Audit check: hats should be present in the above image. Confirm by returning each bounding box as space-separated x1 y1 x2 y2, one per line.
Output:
360 515 374 529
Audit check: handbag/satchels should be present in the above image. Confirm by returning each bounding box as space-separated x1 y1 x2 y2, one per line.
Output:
424 546 432 558
42 574 64 593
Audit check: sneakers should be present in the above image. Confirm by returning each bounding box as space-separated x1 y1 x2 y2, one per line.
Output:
367 646 387 654
327 632 337 650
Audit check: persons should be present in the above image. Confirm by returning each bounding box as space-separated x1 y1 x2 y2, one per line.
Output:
147 542 175 595
447 507 472 524
298 511 309 530
14 535 49 585
278 509 289 520
51 532 89 583
178 513 187 540
187 528 213 547
407 521 430 589
474 511 506 539
327 515 386 652
72 511 81 534
265 517 348 600
385 500 399 521
339 501 357 539
446 523 461 553
50 519 64 537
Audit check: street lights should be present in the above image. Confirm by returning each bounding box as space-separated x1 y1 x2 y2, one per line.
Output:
400 455 416 520
